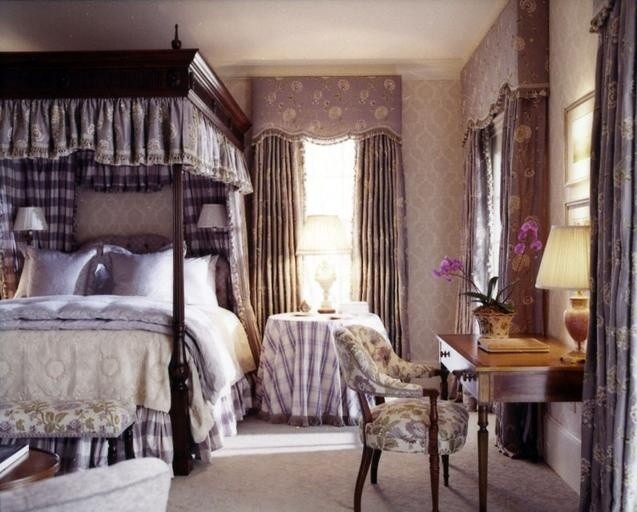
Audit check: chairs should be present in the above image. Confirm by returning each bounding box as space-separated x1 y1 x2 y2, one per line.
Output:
1 457 172 511
336 326 470 505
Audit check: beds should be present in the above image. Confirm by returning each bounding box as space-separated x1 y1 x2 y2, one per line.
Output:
3 23 258 479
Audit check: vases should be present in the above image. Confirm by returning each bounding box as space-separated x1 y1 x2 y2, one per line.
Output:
473 306 512 338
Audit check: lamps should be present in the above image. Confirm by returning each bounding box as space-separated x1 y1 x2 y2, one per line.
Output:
196 203 233 239
10 206 53 257
534 225 595 365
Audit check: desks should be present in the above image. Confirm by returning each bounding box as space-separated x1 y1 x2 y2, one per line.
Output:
438 330 606 507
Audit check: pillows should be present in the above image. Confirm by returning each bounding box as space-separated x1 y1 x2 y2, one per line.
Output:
108 243 184 300
184 253 213 304
210 253 221 312
13 246 98 298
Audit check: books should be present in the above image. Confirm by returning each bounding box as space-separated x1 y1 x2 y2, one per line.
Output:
0 443 30 472
478 337 549 352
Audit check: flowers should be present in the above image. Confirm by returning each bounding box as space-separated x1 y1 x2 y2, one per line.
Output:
433 218 542 312
